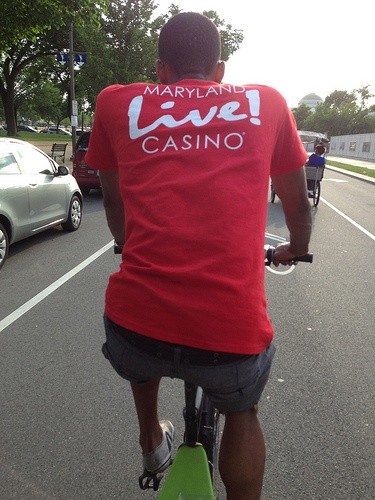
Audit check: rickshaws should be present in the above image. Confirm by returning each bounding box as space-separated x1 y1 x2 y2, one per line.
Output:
269 128 330 207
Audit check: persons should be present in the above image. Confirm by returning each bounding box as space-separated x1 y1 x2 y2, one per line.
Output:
307 144 326 168
83 11 313 500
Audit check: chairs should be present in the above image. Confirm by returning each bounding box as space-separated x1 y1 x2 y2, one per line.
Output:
45 143 68 163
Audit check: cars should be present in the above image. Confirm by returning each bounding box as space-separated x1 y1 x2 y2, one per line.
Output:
0 138 86 267
71 131 102 195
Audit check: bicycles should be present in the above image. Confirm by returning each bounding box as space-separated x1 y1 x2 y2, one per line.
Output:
111 240 315 500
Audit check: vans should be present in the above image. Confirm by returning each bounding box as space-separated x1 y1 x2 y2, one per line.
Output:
47 127 73 137
17 125 39 134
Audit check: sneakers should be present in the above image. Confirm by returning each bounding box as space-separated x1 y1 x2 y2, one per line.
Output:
142 419 175 475
